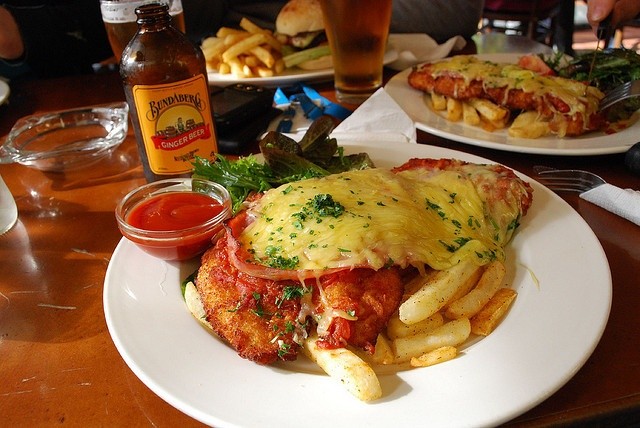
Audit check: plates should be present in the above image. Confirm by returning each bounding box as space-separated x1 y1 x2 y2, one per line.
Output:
385 54 636 153
101 140 614 426
207 51 400 85
0 99 130 174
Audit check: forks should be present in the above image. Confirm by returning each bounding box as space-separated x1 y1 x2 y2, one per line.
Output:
538 169 609 195
601 81 640 110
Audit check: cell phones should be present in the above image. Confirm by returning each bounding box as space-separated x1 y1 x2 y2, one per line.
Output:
211 83 266 125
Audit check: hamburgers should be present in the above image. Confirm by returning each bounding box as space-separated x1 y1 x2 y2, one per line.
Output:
275 1 332 69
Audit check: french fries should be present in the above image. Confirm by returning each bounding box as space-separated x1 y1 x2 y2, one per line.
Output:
299 244 519 402
429 93 551 136
196 18 287 78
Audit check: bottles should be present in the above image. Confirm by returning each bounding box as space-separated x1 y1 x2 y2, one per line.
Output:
121 2 225 178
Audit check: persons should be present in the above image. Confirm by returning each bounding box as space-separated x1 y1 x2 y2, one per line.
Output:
588 1 640 56
184 0 486 44
0 0 31 67
